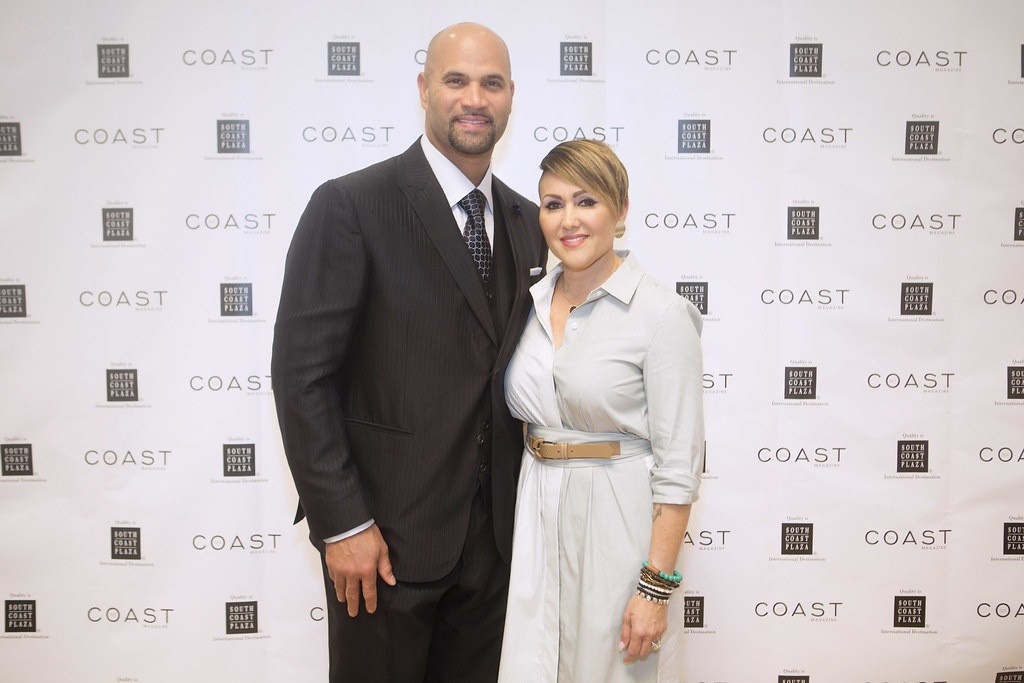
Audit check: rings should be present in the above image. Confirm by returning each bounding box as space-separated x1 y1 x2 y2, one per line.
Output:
651 641 661 650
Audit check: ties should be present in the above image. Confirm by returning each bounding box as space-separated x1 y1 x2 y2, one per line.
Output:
457 189 493 290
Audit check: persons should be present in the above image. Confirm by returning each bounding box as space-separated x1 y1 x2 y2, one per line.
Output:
497 138 704 683
273 22 549 683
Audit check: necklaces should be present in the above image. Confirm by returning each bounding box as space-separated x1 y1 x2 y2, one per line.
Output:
569 305 577 313
636 561 682 605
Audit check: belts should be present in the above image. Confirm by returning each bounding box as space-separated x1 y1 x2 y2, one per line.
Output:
524 431 622 461
